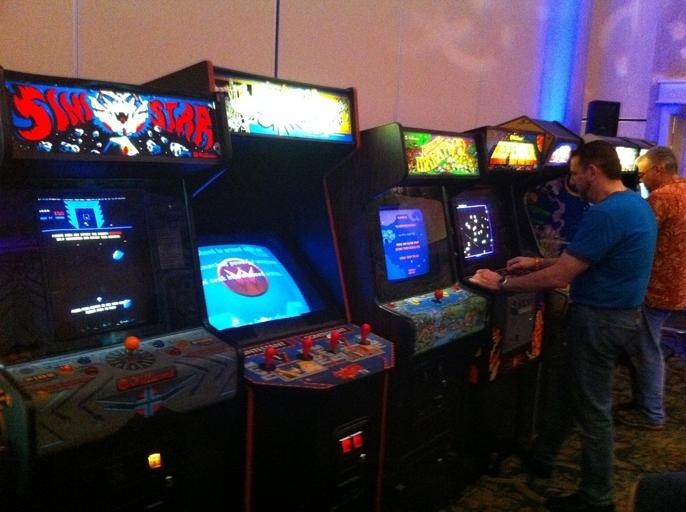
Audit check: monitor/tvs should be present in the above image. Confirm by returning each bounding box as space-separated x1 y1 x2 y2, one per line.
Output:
188 228 325 332
29 180 156 339
452 195 500 264
376 207 434 282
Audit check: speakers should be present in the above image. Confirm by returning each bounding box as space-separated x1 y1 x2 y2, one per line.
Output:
585 100 621 137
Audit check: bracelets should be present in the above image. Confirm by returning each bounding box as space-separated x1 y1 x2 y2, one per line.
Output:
536 257 543 270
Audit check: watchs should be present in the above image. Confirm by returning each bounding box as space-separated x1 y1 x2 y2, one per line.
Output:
498 275 509 293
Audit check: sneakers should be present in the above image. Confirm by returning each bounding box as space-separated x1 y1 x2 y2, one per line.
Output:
482 458 549 483
546 492 616 512
615 408 664 430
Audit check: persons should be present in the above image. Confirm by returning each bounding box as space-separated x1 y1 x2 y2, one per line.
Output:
468 138 659 511
617 144 686 430
